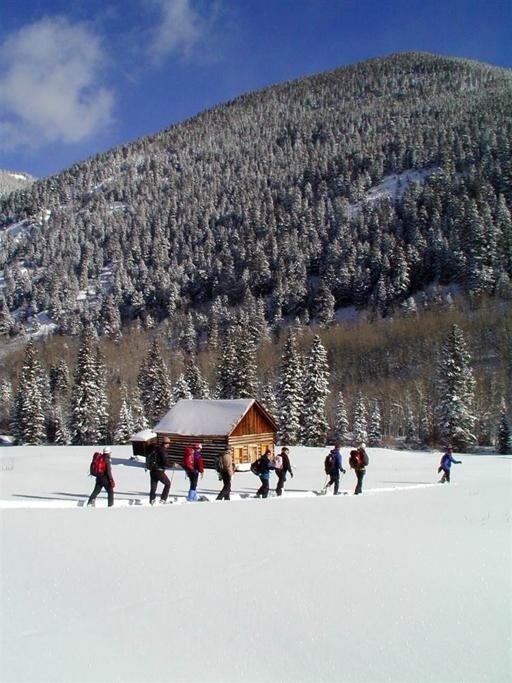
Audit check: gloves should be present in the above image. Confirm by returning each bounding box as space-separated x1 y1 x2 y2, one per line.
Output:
109 479 115 487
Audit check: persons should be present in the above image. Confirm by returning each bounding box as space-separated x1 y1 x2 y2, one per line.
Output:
274 447 294 495
349 442 369 495
87 447 115 507
180 442 204 501
253 449 274 498
439 445 461 483
216 444 234 500
321 442 345 495
146 436 179 505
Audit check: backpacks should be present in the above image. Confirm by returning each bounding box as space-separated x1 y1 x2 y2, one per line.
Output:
90 452 107 477
325 454 337 474
146 444 162 470
184 447 195 473
215 456 224 472
349 450 363 468
272 455 283 470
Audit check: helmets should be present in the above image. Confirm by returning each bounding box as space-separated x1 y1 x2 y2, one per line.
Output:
103 447 112 454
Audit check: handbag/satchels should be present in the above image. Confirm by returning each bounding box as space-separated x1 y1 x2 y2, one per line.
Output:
251 460 263 475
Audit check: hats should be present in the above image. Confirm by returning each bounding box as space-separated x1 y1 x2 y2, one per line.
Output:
360 442 366 448
195 443 202 449
163 437 170 443
